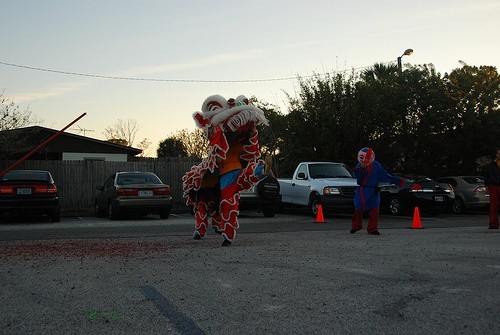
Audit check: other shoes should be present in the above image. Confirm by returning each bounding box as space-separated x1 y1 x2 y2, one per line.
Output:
350 225 362 233
368 230 380 235
263 154 273 175
488 226 499 229
194 234 201 240
221 240 231 246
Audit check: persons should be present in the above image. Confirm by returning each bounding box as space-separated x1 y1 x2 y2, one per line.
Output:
215 142 272 246
486 149 500 229
193 174 223 239
350 148 410 235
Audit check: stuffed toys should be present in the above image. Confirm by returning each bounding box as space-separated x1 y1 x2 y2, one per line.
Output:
183 95 273 246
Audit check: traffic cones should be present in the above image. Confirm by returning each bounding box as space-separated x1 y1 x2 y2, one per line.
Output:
312 205 327 223
408 207 424 229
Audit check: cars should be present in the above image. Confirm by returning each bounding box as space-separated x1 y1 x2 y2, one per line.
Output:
380 175 455 216
0 170 60 224
94 171 173 219
434 175 491 215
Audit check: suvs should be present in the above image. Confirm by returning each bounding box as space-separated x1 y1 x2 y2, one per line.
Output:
239 164 282 218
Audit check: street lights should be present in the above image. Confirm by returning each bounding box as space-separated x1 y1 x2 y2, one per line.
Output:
397 48 414 176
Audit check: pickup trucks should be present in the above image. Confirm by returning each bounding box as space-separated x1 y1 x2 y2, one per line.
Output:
278 161 360 218
